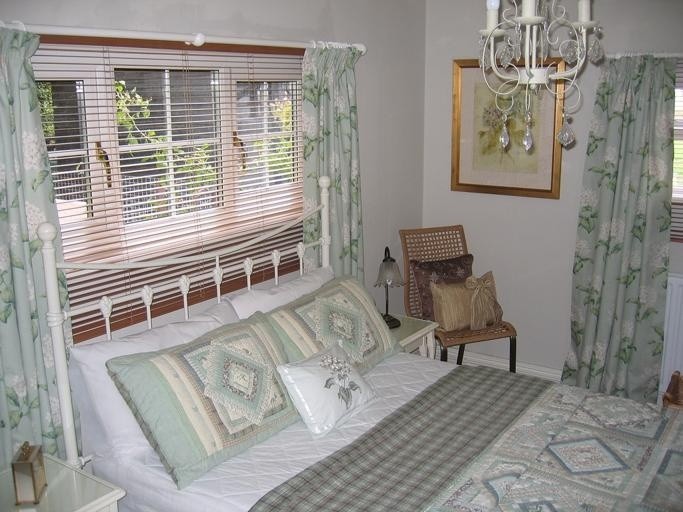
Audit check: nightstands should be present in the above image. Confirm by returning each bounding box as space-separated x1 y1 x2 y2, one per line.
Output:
380 314 440 359
0 453 126 512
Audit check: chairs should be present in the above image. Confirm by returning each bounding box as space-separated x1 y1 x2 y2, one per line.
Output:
398 221 517 371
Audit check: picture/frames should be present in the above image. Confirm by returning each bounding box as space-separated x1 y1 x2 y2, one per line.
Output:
451 58 567 199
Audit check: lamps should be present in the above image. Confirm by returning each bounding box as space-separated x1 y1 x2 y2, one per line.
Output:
69 300 250 469
374 246 401 333
478 1 603 153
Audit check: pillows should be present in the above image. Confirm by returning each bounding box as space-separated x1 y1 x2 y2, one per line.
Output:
409 254 476 320
277 344 378 436
232 267 336 318
263 275 405 397
431 271 502 330
105 311 300 489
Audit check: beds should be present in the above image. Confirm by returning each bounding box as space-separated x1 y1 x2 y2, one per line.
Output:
17 177 682 512
